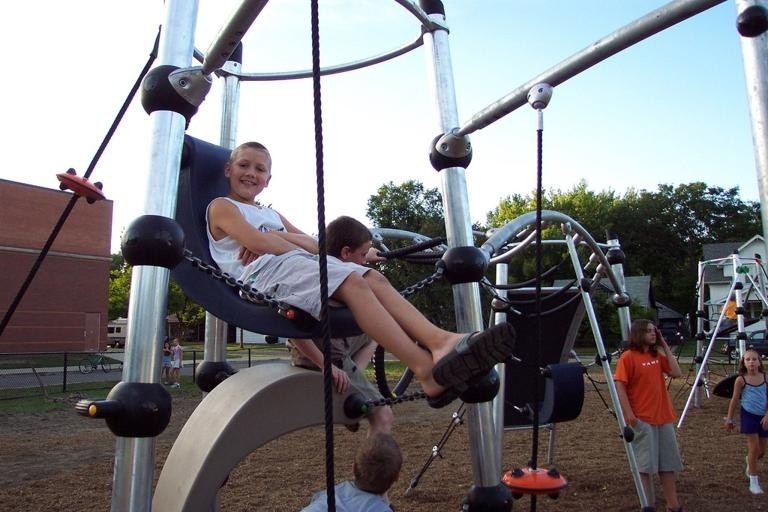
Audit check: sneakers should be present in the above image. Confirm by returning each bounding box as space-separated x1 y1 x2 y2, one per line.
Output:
164 382 180 387
745 454 764 494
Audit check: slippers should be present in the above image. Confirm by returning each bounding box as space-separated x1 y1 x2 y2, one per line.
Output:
420 323 515 409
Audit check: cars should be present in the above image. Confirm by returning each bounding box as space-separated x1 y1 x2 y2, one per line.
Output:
727 329 768 360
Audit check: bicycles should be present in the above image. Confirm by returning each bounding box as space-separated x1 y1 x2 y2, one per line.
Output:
78 347 114 374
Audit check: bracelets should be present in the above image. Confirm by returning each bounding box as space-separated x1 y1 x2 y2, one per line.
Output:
664 347 670 355
725 417 735 425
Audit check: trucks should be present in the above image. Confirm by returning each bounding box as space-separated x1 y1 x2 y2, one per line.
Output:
105 318 128 347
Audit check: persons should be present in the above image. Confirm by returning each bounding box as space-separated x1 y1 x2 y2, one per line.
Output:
612 319 686 512
296 432 403 512
169 337 184 389
204 141 519 409
161 341 172 385
723 348 767 496
284 216 397 512
